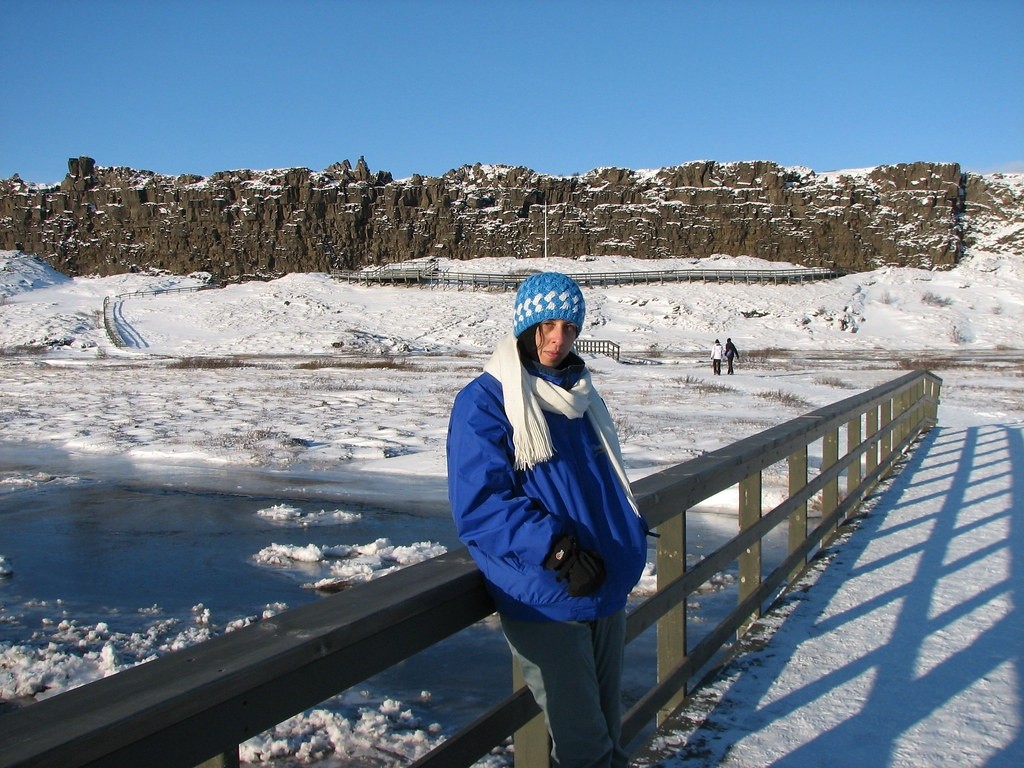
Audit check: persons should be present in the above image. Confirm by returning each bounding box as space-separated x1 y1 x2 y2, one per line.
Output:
446 272 650 767
725 338 739 375
711 339 723 375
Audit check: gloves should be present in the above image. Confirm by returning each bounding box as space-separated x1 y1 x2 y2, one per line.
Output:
544 537 607 597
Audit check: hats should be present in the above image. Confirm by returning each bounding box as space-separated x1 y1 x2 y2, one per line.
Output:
512 272 584 338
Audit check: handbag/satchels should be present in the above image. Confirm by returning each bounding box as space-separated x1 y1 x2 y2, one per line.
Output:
726 348 732 358
